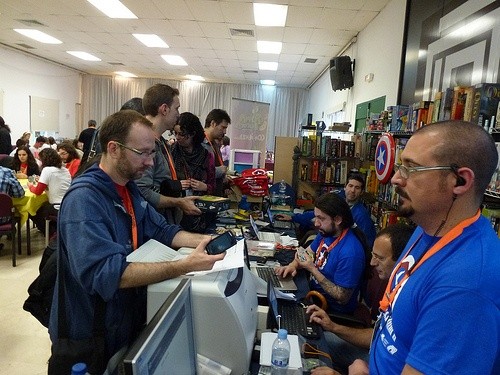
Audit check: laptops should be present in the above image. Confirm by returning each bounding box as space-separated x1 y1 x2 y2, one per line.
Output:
250 214 282 242
266 282 322 339
241 227 297 293
263 203 296 228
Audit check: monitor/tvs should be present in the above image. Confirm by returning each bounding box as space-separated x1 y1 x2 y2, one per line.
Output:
123 279 198 375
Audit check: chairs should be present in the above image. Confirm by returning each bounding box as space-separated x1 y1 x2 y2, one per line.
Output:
43 202 61 246
0 192 22 266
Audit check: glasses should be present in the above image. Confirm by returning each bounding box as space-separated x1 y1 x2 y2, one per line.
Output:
59 150 68 156
113 142 157 160
371 251 391 262
393 162 460 179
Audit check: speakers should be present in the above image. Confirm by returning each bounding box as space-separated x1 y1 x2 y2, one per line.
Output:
328 56 353 92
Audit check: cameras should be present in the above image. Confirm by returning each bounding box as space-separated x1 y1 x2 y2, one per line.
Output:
205 229 237 256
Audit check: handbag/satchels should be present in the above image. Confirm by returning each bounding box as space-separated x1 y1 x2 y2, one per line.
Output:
23 242 58 327
238 167 269 197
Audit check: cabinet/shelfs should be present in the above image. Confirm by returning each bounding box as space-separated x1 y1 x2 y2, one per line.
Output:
292 124 360 199
358 113 500 229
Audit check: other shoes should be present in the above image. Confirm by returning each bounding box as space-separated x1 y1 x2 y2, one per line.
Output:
7 227 16 240
50 233 57 241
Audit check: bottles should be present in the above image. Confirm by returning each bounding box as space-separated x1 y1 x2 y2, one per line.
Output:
72 363 90 375
271 329 291 375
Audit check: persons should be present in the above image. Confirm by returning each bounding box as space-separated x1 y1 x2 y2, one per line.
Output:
78 119 97 158
0 130 83 243
121 83 242 229
348 120 500 375
0 116 12 154
46 110 226 375
304 223 414 375
274 194 365 307
276 174 376 249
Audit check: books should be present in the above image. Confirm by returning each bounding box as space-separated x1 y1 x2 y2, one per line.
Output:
301 159 348 183
486 141 500 194
364 200 418 236
367 105 412 133
394 137 409 168
352 163 402 205
481 200 500 241
328 121 351 132
411 82 500 128
303 134 364 158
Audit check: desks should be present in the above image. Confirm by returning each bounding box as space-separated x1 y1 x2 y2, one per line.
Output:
205 200 340 375
12 177 49 255
